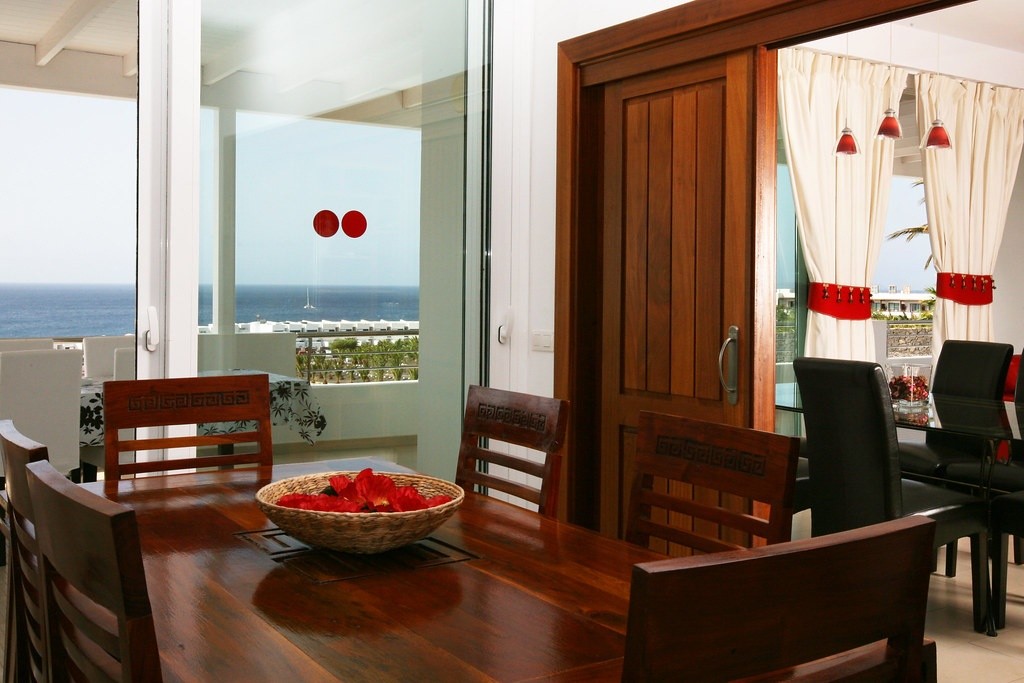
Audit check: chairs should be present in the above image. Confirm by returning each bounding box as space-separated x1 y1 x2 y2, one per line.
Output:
0 417 58 682
900 341 1013 576
624 408 801 544
947 348 1024 578
78 347 138 485
454 382 571 521
0 348 85 566
618 513 938 683
1 335 55 353
81 333 136 380
104 374 277 474
793 352 989 635
25 457 162 683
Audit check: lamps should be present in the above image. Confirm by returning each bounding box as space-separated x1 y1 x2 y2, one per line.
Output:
876 24 903 141
832 32 862 158
918 12 956 150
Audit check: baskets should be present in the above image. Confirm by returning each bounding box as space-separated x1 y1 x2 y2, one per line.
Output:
255 470 464 556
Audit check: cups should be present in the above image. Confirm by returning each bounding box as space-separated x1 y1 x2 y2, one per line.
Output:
885 362 932 414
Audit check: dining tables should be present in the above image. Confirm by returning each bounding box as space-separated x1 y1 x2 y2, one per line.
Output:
775 379 1023 629
2 454 934 683
79 370 333 482
